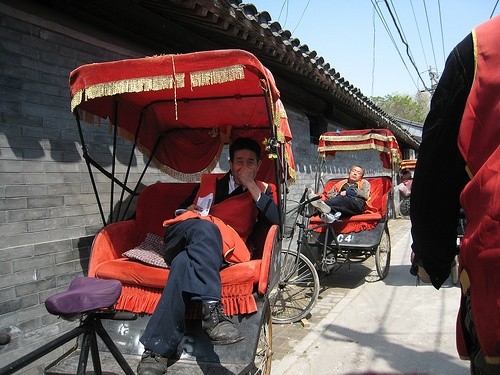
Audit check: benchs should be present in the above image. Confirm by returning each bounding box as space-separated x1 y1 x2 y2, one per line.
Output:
88 183 280 317
307 176 392 234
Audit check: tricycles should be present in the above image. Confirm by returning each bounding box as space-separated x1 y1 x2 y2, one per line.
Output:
266 128 419 325
1 49 298 374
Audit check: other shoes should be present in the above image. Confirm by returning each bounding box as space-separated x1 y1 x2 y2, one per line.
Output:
306 188 315 215
307 194 332 214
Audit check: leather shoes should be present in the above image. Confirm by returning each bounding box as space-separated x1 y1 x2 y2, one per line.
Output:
136 348 169 375
200 301 245 345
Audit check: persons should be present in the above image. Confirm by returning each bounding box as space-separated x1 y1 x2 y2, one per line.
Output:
137 137 286 375
410 14 500 375
397 170 413 197
306 165 370 216
